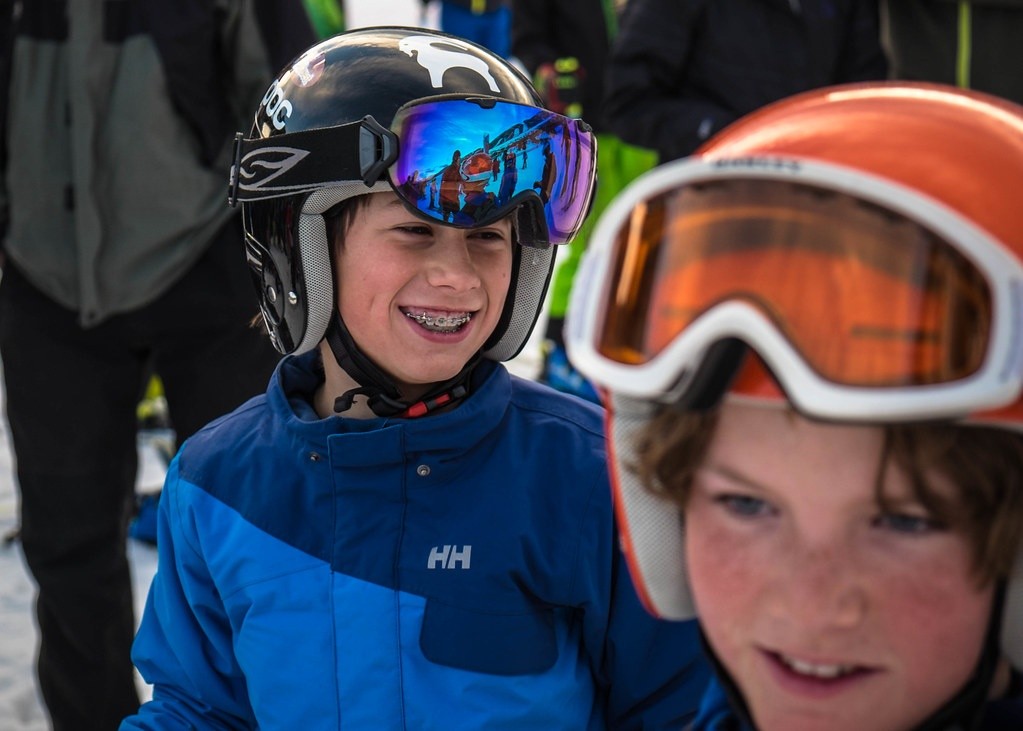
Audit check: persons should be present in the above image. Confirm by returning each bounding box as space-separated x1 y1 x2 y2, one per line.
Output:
440 150 462 221
454 154 498 225
401 150 436 209
533 142 557 207
68 26 728 731
598 0 890 168
563 81 1023 731
0 0 318 731
522 150 528 169
423 0 608 124
492 156 500 182
498 150 518 204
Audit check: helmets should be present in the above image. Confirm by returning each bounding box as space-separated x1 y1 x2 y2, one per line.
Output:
227 25 557 361
596 82 1023 666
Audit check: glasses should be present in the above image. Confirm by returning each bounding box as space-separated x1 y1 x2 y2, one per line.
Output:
383 92 599 249
562 149 1023 427
